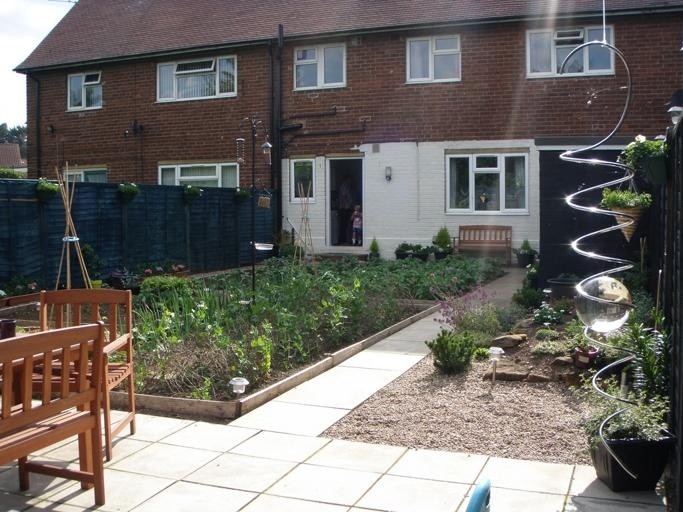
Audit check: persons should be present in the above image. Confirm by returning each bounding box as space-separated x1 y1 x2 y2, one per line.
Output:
350 204 362 246
338 174 352 244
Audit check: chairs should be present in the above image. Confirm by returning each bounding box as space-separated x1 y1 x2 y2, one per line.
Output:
0 289 135 505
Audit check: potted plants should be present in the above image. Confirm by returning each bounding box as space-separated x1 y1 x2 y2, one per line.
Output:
395 243 433 261
618 134 669 185
575 338 598 368
119 182 139 203
184 184 204 205
234 185 252 203
569 370 678 490
598 187 652 244
432 226 452 260
369 237 380 261
518 238 535 268
37 176 62 202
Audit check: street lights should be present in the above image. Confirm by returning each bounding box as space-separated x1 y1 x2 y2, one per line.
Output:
248 114 273 291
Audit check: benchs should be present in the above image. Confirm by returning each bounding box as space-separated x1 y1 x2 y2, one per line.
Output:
454 225 512 264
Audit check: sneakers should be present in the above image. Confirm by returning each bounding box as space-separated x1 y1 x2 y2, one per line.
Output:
351 243 361 246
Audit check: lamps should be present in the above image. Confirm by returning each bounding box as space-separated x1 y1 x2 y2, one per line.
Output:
385 167 392 181
